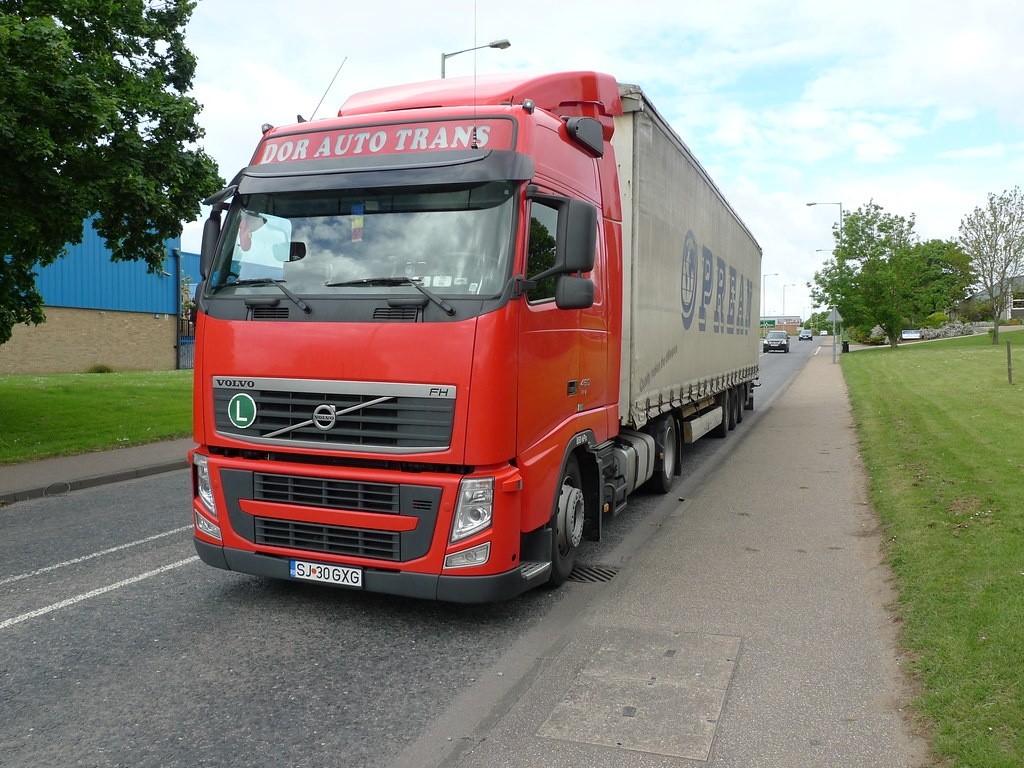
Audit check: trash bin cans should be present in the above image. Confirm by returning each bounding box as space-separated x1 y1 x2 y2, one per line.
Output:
842 340 850 353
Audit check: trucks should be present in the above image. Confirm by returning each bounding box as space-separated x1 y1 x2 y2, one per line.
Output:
187 70 762 605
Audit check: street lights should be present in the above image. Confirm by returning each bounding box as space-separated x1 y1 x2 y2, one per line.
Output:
764 274 778 337
815 249 838 252
806 202 842 346
783 284 795 330
440 39 511 79
803 306 809 327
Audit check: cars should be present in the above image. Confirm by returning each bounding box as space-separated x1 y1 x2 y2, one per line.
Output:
763 331 790 353
820 331 827 335
799 330 812 341
